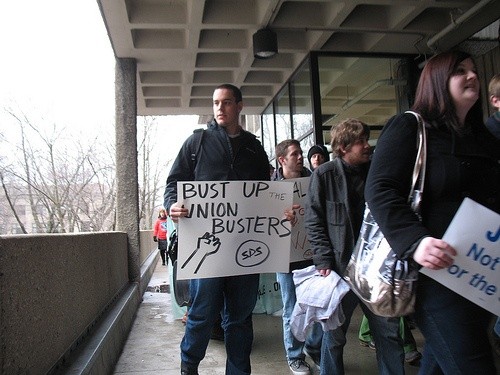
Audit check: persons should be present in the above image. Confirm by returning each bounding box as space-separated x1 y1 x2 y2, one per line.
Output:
364 49 500 375
359 315 419 363
308 144 330 173
303 118 405 375
163 84 300 375
271 139 326 375
153 209 168 267
484 72 500 136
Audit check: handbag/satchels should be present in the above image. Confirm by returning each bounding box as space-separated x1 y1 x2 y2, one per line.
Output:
344 110 426 319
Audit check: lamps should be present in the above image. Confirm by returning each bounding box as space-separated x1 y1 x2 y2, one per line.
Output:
251 24 278 60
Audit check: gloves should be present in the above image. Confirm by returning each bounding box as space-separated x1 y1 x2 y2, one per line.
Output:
153 237 157 242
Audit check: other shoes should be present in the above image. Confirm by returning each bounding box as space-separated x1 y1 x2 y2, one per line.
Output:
288 360 312 375
162 263 165 265
361 340 375 349
405 351 422 363
